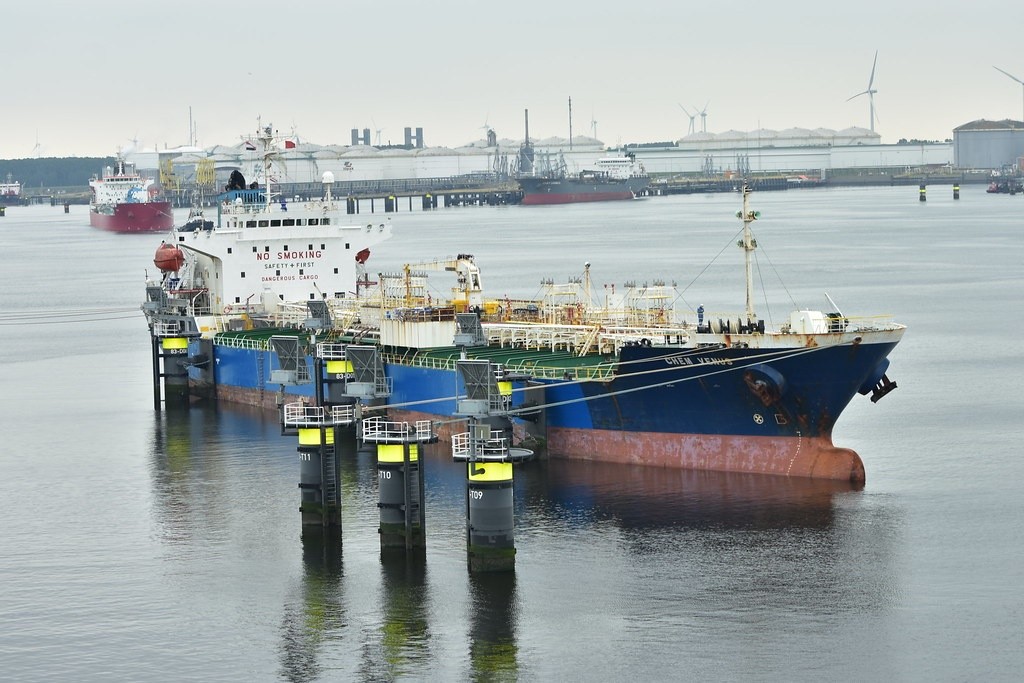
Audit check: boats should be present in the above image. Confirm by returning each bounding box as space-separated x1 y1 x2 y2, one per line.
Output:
514 170 638 205
0 170 21 197
86 144 176 234
138 174 911 485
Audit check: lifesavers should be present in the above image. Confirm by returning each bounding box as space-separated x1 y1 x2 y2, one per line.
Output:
224 307 230 314
